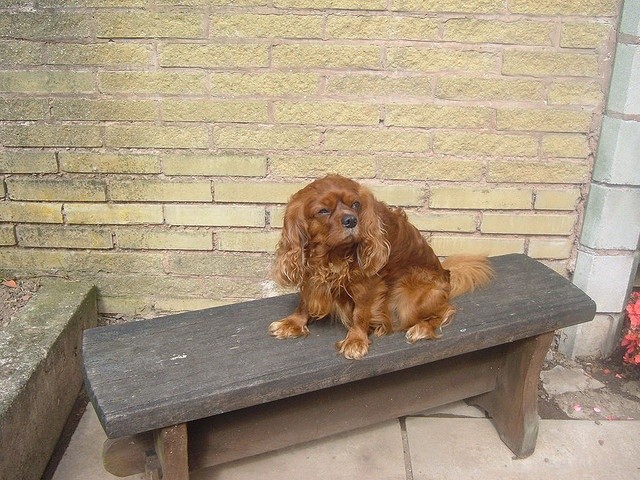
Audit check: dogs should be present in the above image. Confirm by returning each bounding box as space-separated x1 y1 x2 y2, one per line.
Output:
268 172 496 360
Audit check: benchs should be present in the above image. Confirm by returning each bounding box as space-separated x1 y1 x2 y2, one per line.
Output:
78 251 597 480
